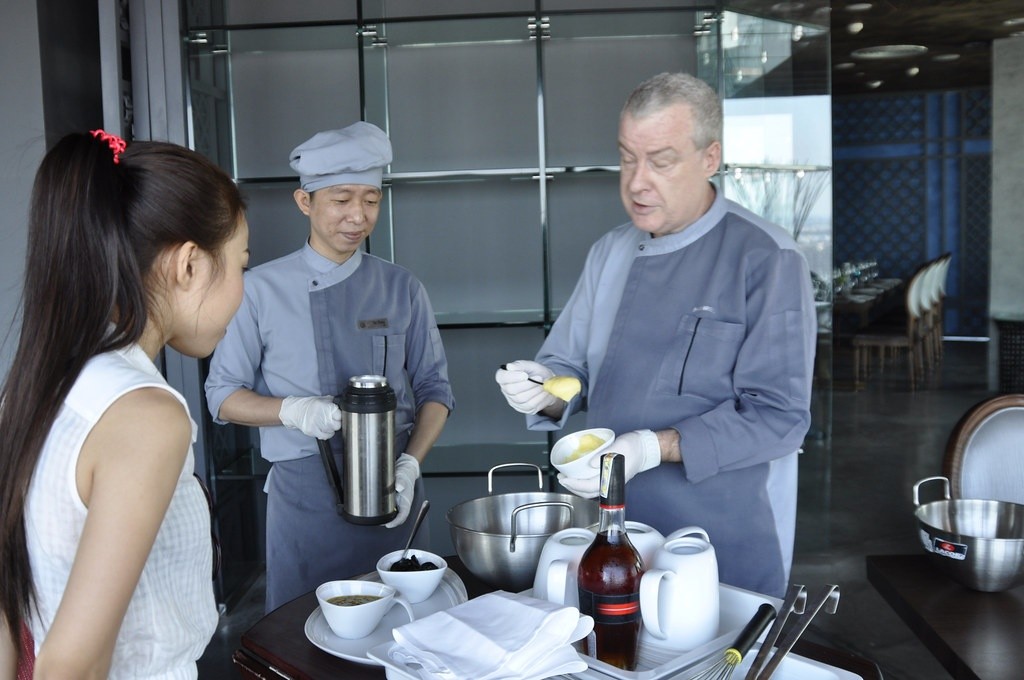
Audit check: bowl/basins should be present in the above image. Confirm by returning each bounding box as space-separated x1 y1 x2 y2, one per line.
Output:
550 428 615 478
316 580 396 639
376 549 447 604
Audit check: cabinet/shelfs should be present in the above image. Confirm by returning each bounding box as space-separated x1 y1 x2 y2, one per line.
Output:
180 0 833 616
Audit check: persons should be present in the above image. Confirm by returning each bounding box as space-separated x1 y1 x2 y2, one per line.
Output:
205 121 456 613
495 71 816 597
0 129 253 680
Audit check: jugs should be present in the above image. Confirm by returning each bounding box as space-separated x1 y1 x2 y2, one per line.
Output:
317 375 398 525
639 527 719 652
532 528 597 612
624 521 710 571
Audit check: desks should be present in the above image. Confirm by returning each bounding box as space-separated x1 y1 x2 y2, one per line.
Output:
231 554 883 680
865 555 1024 680
832 278 902 388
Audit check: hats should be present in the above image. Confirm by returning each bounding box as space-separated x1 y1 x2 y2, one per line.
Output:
289 121 393 193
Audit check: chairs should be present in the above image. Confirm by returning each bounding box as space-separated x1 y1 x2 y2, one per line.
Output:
851 252 952 394
941 392 1024 505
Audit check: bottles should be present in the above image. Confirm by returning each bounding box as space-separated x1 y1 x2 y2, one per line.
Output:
578 453 642 673
833 258 880 293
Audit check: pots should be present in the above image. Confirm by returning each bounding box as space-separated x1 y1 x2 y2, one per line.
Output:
913 475 1023 592
446 463 599 593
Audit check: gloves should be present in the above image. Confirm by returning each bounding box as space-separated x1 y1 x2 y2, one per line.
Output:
496 360 557 414
279 396 342 440
557 429 661 498
379 452 419 528
